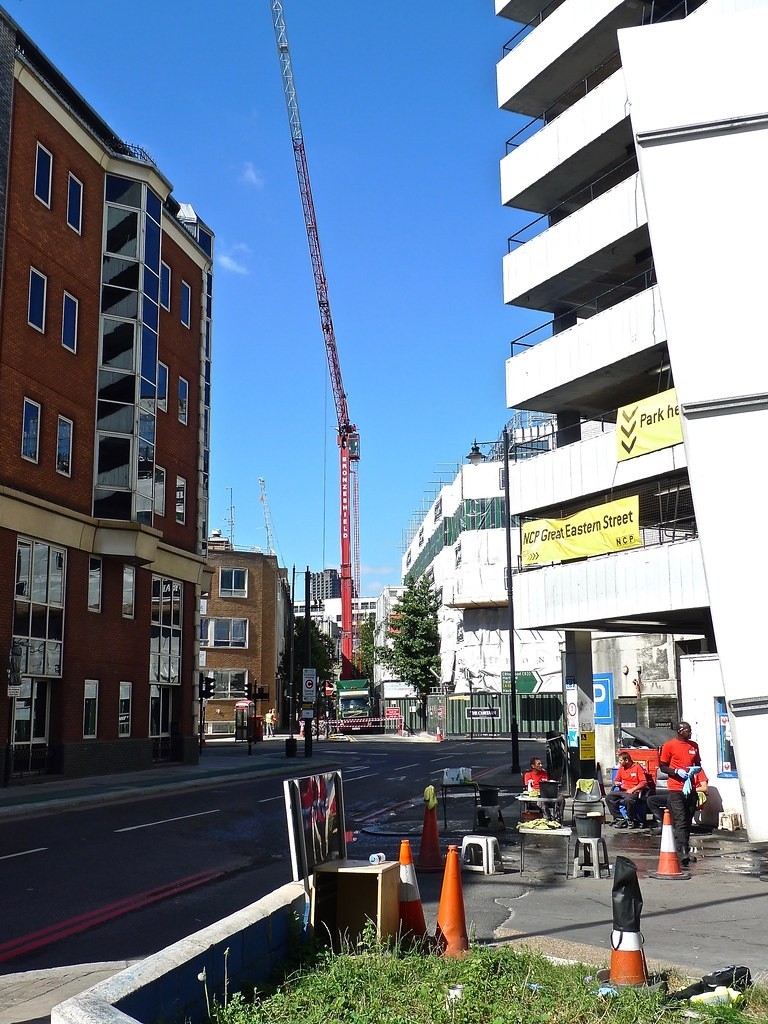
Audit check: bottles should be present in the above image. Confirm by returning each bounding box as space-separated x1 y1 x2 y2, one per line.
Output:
369 853 386 865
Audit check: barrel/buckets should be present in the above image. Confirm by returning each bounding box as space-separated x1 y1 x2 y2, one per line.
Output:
573 813 602 838
539 781 559 798
479 787 499 806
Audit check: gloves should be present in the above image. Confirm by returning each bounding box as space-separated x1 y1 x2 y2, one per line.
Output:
675 769 688 779
683 778 693 796
686 766 700 777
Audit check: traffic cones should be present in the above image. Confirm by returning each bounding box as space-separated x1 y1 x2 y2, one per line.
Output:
648 809 692 881
395 839 432 948
608 884 650 988
433 843 476 960
200 731 207 746
436 724 443 741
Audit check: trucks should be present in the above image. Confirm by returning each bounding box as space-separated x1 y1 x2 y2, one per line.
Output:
328 695 335 719
333 679 374 734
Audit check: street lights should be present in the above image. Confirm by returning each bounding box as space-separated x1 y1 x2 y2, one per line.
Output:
466 425 520 774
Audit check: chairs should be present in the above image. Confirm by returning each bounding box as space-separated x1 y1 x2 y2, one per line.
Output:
608 786 651 828
520 769 545 816
571 779 608 830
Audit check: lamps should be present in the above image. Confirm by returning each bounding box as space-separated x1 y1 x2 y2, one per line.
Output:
636 666 642 672
622 665 628 676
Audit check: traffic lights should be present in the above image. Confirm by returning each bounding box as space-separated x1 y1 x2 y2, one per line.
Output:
205 677 215 698
244 683 252 700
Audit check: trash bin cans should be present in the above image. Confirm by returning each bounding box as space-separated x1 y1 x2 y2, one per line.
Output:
573 813 602 838
478 788 499 807
538 780 559 808
286 739 297 758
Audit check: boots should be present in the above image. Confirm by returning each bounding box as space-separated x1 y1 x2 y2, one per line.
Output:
680 847 697 866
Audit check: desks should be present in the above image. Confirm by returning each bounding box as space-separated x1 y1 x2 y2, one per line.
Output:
518 796 565 826
438 782 478 830
519 827 573 880
311 858 399 951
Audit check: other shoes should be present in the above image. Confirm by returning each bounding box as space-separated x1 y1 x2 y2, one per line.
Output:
628 820 635 829
650 826 662 835
610 818 624 826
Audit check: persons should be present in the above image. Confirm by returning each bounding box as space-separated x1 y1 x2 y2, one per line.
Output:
272 708 277 735
646 762 709 840
524 758 566 821
265 709 275 738
659 722 701 867
605 752 648 828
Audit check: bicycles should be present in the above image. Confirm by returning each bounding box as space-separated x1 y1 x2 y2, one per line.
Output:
312 719 337 736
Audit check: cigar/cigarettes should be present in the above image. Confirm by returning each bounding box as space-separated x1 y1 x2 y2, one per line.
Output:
542 768 545 771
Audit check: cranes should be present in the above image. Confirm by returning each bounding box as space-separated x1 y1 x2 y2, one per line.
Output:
268 0 360 678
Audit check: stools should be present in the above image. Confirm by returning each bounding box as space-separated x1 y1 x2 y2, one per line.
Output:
718 812 743 833
572 837 611 880
460 835 504 877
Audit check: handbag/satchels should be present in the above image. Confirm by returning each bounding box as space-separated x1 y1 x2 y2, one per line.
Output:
610 856 645 950
